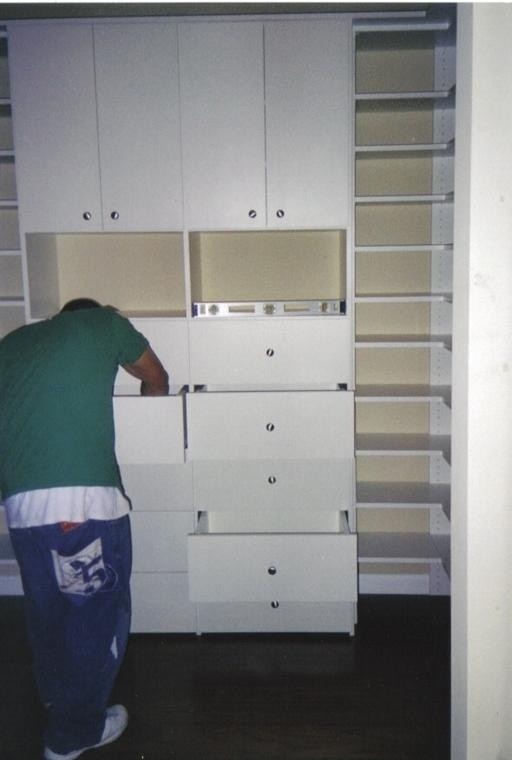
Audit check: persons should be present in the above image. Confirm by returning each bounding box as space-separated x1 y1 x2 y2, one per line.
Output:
0 297 170 760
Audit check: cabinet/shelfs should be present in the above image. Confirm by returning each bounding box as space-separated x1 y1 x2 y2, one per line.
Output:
1 7 459 636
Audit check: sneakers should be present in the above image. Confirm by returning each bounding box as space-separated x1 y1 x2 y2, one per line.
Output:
43 703 129 760
